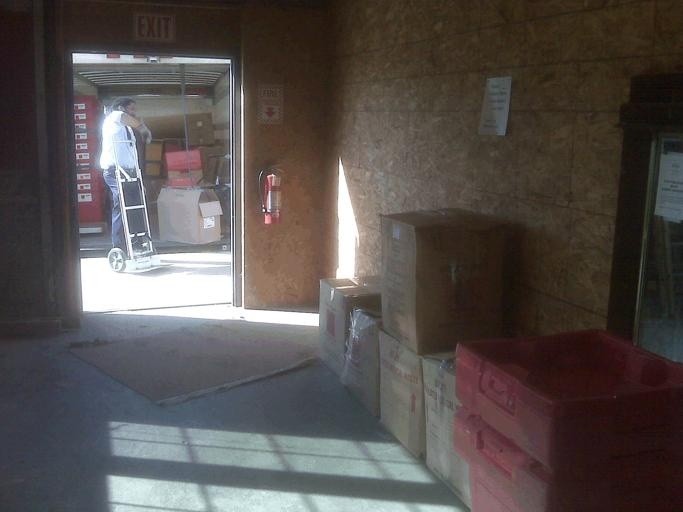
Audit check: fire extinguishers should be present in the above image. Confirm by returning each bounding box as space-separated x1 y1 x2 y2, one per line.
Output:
258 165 285 225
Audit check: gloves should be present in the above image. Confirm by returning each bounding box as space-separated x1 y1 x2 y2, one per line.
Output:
135 122 152 147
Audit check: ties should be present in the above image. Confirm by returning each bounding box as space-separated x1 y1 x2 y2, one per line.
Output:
124 125 132 146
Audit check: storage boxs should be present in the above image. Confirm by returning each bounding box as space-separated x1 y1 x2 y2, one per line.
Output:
455 329 682 512
316 275 381 409
421 351 471 504
382 211 516 354
379 327 423 456
134 112 229 247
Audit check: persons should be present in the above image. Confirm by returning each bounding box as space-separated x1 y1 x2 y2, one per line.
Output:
99 96 152 258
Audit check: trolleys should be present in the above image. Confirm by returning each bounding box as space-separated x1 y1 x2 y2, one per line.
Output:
109 123 155 271
